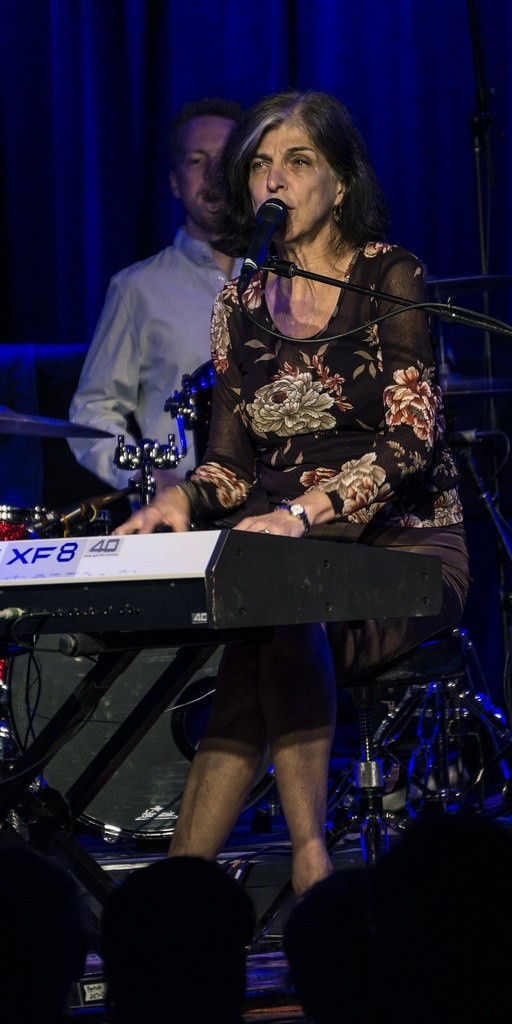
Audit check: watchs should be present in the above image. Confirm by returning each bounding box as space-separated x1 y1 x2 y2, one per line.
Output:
279 503 310 533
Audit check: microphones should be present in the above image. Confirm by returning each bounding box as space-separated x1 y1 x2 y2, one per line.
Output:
458 430 491 457
236 198 289 295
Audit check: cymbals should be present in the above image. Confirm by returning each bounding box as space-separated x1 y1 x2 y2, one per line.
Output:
426 272 512 294
0 408 118 439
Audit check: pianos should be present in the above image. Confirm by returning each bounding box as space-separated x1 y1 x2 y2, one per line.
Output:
1 528 446 634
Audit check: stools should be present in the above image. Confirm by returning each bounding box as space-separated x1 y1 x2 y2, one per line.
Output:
251 635 466 946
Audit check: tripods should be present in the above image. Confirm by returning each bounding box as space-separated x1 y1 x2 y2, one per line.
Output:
247 675 512 951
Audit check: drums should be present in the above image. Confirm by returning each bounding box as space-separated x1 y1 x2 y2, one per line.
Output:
7 631 279 842
0 505 55 695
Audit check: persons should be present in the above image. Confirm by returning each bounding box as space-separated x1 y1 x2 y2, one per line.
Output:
0 845 92 1023
281 869 369 1024
68 96 255 532
369 814 512 1024
101 858 257 1024
112 92 470 900
